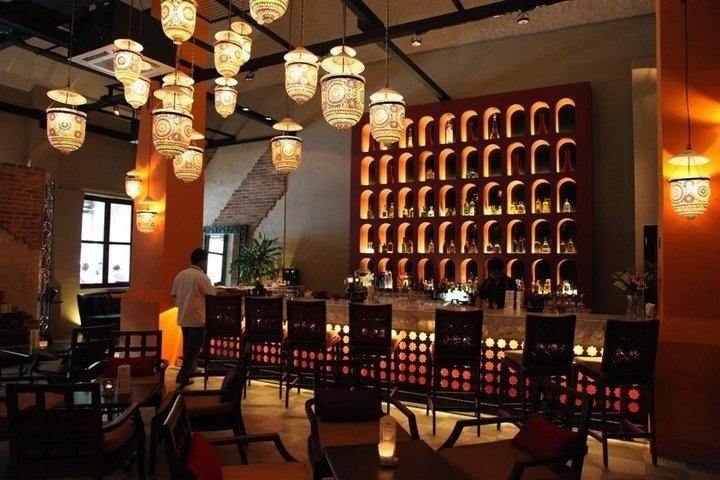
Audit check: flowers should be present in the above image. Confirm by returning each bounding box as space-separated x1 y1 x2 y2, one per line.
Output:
612 268 649 303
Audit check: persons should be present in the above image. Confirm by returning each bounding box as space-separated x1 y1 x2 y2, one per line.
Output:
475 256 518 309
171 248 217 386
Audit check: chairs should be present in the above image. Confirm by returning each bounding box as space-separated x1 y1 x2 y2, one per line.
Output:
346 301 402 416
202 292 247 396
282 298 340 408
240 295 285 400
573 317 660 468
425 309 482 436
495 313 576 430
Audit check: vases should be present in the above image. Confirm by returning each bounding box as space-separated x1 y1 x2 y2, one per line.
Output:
626 301 641 320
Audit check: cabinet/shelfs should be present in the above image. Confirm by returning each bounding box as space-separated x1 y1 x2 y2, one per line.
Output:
347 81 594 311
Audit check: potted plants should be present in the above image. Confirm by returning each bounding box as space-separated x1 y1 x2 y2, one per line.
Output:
228 231 283 297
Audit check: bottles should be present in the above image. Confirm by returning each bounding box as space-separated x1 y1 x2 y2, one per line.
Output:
266 277 295 297
364 113 573 311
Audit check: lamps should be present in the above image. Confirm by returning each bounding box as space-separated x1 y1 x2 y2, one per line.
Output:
44 0 407 237
662 1 711 219
